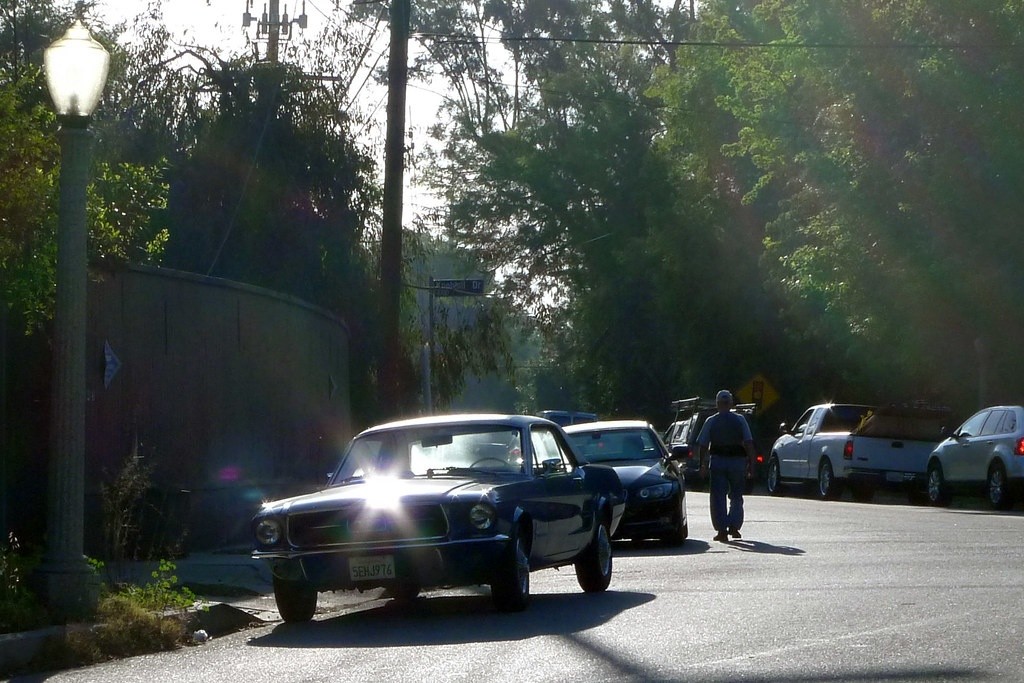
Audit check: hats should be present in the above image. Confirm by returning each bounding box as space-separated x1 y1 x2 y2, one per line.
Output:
716 390 733 401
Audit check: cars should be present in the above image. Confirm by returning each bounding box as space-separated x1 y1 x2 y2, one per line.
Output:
559 419 690 547
250 412 629 624
925 406 1024 512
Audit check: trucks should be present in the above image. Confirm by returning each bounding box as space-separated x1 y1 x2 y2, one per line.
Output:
660 396 770 496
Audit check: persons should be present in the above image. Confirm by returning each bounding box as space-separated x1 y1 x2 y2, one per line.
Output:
696 390 754 542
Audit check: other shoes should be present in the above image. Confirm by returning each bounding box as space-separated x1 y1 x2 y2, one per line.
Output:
714 530 728 543
729 526 741 538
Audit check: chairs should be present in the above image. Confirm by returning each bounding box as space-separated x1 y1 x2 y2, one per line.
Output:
622 434 644 460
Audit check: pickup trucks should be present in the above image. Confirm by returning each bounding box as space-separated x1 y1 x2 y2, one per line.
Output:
766 403 940 504
